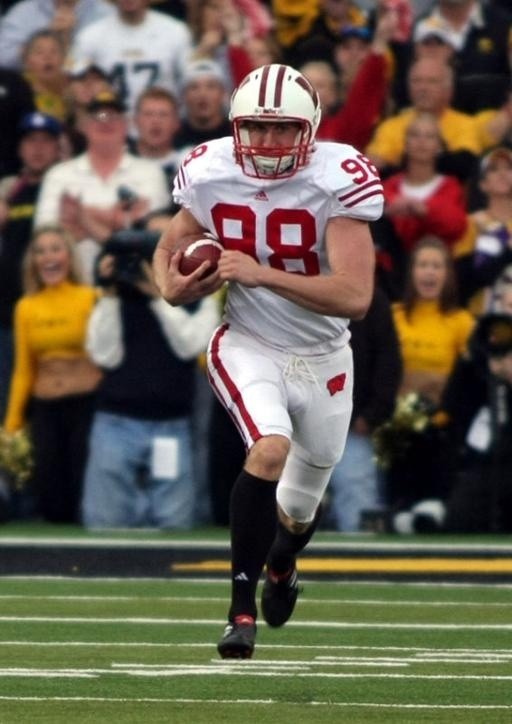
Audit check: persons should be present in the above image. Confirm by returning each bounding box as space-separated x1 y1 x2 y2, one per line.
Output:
1 1 511 533
150 62 385 660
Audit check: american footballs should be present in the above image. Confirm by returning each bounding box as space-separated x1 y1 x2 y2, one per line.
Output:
169 232 225 280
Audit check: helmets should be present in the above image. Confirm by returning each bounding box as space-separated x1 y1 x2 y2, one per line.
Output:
229 64 321 178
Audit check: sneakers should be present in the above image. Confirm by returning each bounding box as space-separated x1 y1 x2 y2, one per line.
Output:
218 615 256 658
261 563 297 626
